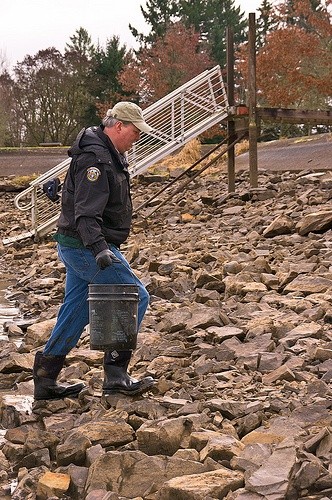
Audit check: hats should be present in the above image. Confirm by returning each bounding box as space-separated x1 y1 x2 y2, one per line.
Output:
106 102 153 134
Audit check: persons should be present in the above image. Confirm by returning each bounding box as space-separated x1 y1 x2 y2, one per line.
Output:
33 101 152 401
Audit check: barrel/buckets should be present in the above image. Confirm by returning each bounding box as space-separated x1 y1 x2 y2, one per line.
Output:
87 261 142 349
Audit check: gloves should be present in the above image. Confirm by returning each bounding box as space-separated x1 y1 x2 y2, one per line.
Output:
96 250 122 270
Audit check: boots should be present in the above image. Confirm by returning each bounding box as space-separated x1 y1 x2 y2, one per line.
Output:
102 351 155 394
33 351 87 400
